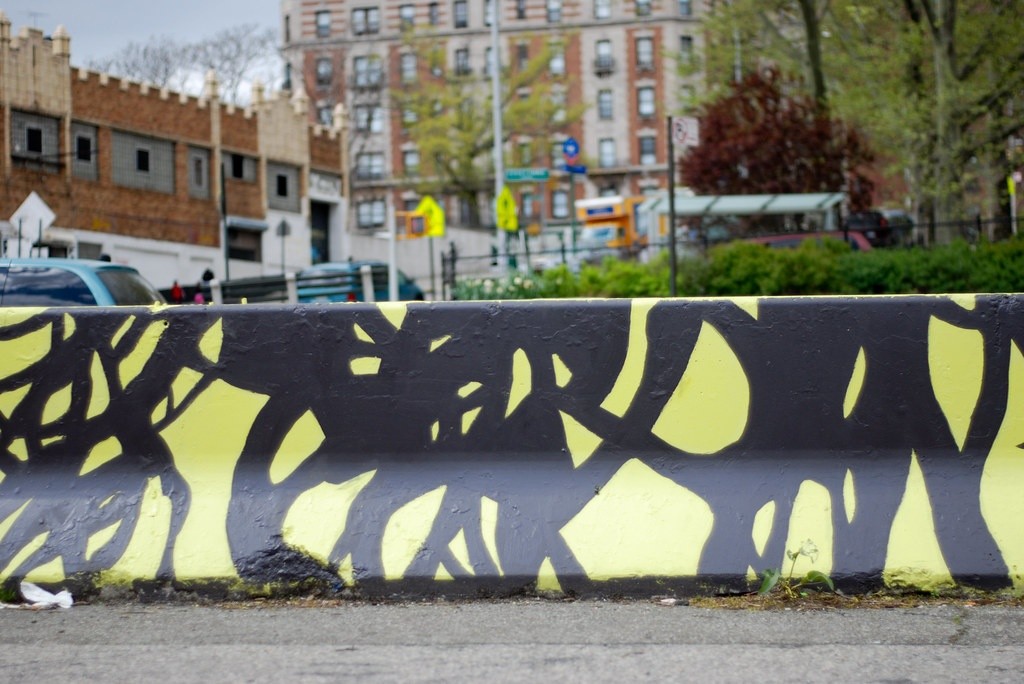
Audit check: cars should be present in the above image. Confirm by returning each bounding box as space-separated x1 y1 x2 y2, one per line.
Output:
0 258 168 306
294 256 425 301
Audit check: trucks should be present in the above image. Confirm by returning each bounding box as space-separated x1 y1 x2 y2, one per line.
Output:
575 195 669 261
